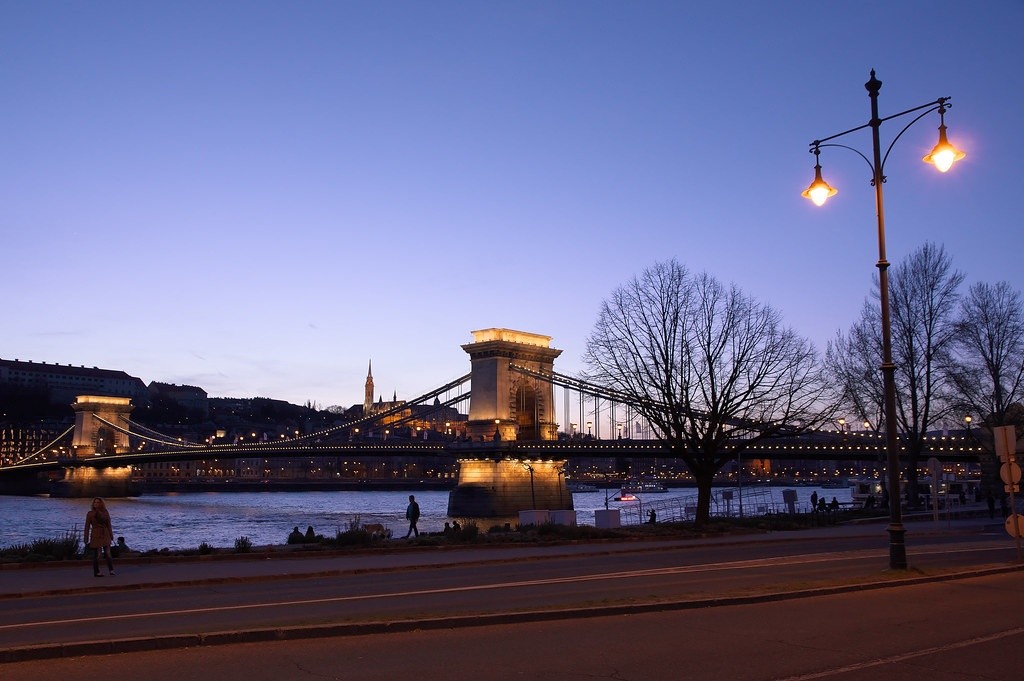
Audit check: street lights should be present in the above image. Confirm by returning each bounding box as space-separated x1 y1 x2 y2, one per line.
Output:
802 64 967 574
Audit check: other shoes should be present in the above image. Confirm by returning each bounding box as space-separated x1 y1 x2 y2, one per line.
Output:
94 573 104 577
110 572 117 576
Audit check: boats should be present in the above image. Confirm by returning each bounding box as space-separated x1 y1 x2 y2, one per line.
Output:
620 475 670 493
565 478 600 493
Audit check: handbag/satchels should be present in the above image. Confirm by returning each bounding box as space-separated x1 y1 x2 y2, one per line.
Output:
104 540 120 558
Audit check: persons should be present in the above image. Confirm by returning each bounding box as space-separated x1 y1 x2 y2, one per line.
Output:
83 488 966 578
